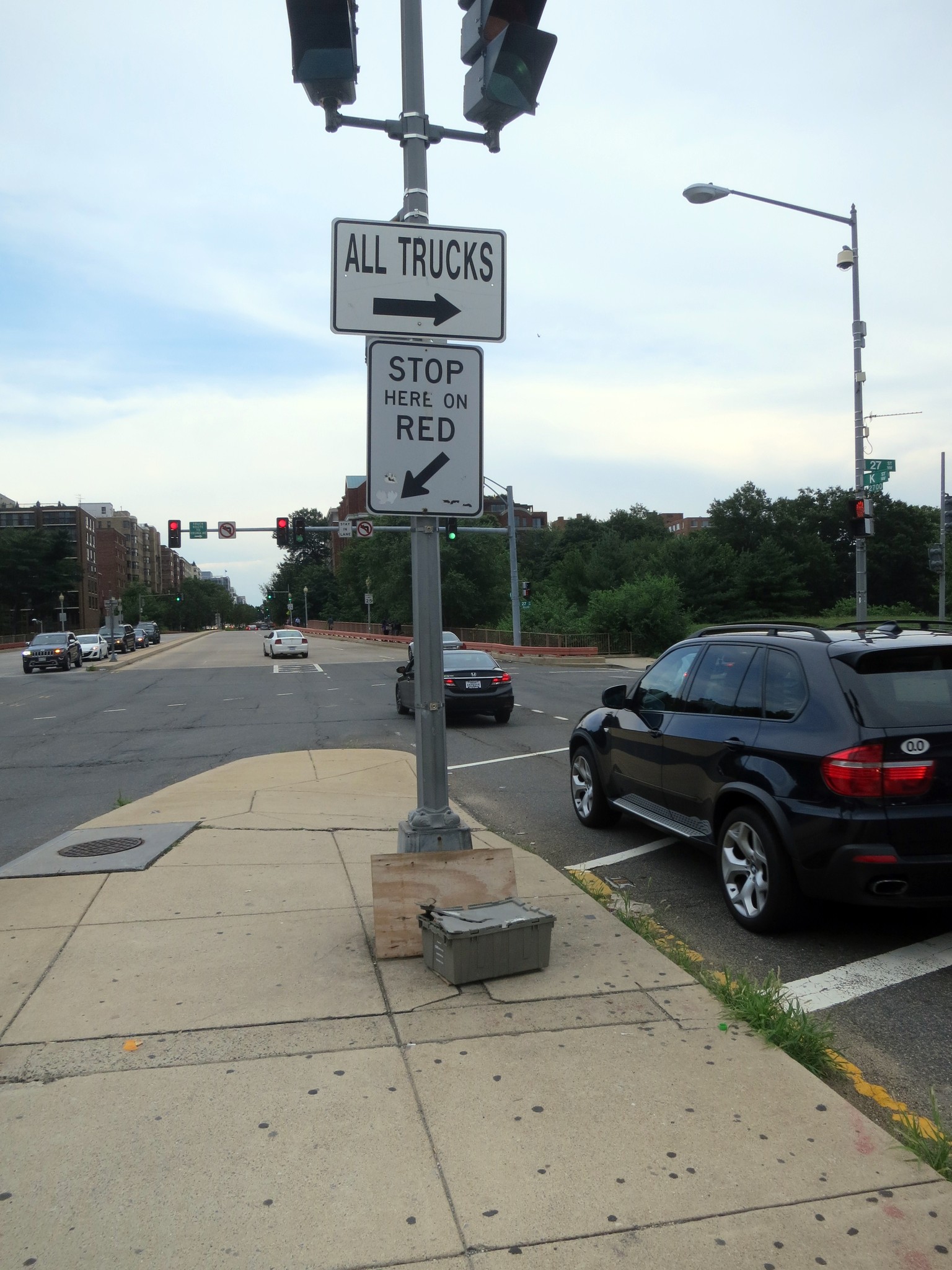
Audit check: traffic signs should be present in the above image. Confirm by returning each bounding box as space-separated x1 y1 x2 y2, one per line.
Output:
188 522 208 539
327 219 509 345
364 341 490 519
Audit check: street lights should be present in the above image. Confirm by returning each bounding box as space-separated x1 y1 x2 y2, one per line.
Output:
303 586 308 628
59 593 65 633
31 618 43 634
118 597 123 624
365 575 371 633
680 184 872 623
288 593 293 626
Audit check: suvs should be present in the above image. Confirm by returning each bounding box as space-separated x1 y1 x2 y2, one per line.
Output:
22 620 160 673
569 621 952 933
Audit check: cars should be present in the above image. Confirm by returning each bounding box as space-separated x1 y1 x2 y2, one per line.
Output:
263 629 309 659
396 630 515 724
204 621 271 631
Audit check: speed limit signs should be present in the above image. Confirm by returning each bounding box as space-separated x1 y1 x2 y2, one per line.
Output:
365 594 373 605
287 604 294 610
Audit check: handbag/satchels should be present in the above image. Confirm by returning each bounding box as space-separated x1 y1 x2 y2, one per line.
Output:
330 625 334 628
386 626 392 631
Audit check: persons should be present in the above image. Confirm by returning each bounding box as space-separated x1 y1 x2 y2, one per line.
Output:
326 615 334 631
286 616 300 627
381 618 401 642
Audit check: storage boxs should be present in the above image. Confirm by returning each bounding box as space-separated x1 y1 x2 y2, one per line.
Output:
415 897 557 985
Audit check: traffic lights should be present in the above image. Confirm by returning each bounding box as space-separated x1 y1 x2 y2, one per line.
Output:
288 1 359 91
267 590 272 599
446 516 459 543
521 581 532 597
176 592 181 602
458 0 555 133
168 519 181 548
276 517 305 547
847 498 871 539
